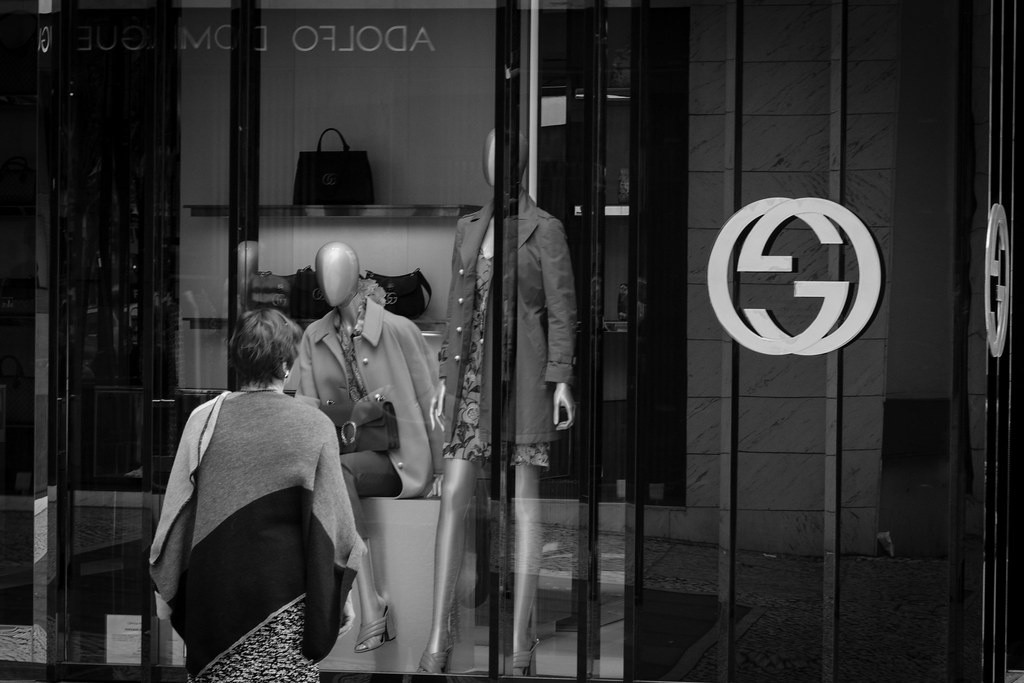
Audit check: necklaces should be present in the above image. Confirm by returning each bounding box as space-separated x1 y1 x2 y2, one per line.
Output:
240 388 278 394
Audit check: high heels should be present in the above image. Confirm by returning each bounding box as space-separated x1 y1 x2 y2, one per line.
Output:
354 605 396 653
513 639 539 677
417 632 454 675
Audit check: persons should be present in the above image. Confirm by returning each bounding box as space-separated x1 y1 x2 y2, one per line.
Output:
150 310 366 683
413 128 578 677
279 242 441 652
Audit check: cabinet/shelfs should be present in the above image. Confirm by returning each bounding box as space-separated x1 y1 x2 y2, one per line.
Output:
574 89 631 334
182 205 488 401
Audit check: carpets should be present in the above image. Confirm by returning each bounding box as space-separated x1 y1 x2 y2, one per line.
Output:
0 514 767 683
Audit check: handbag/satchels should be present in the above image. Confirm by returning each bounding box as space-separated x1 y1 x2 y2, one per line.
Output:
365 268 432 320
247 265 333 320
320 394 400 454
293 127 375 205
